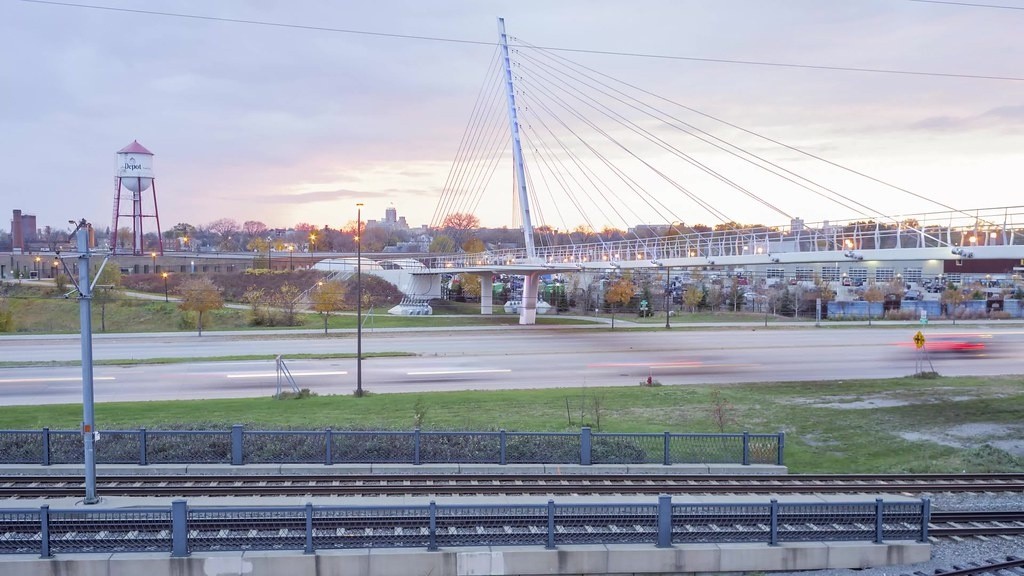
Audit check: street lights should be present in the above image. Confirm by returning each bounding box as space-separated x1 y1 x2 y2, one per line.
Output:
54 262 59 288
151 253 156 274
353 200 365 397
308 234 315 268
289 246 294 269
162 273 169 302
35 257 40 281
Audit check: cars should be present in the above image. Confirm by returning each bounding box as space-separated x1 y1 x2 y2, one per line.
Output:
436 273 1024 319
